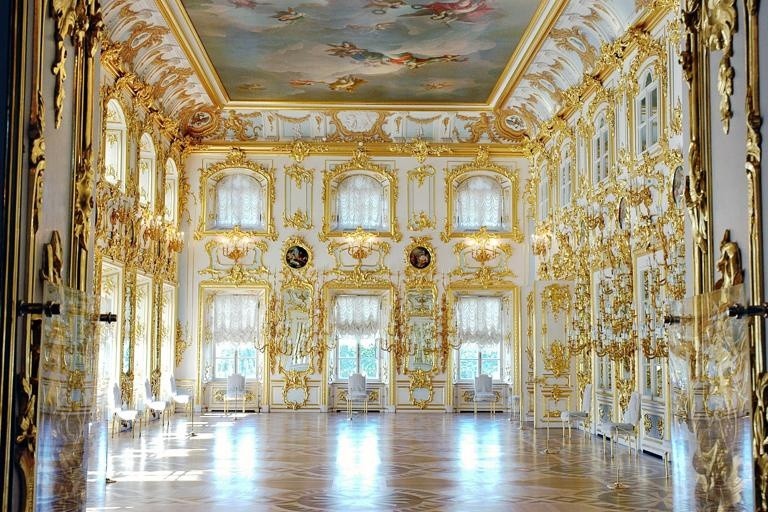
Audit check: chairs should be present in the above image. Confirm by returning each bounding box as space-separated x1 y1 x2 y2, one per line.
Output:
512 394 520 418
346 373 368 414
170 375 192 416
597 391 641 458
144 378 169 426
112 383 141 439
224 373 247 415
560 384 592 441
473 374 496 415
